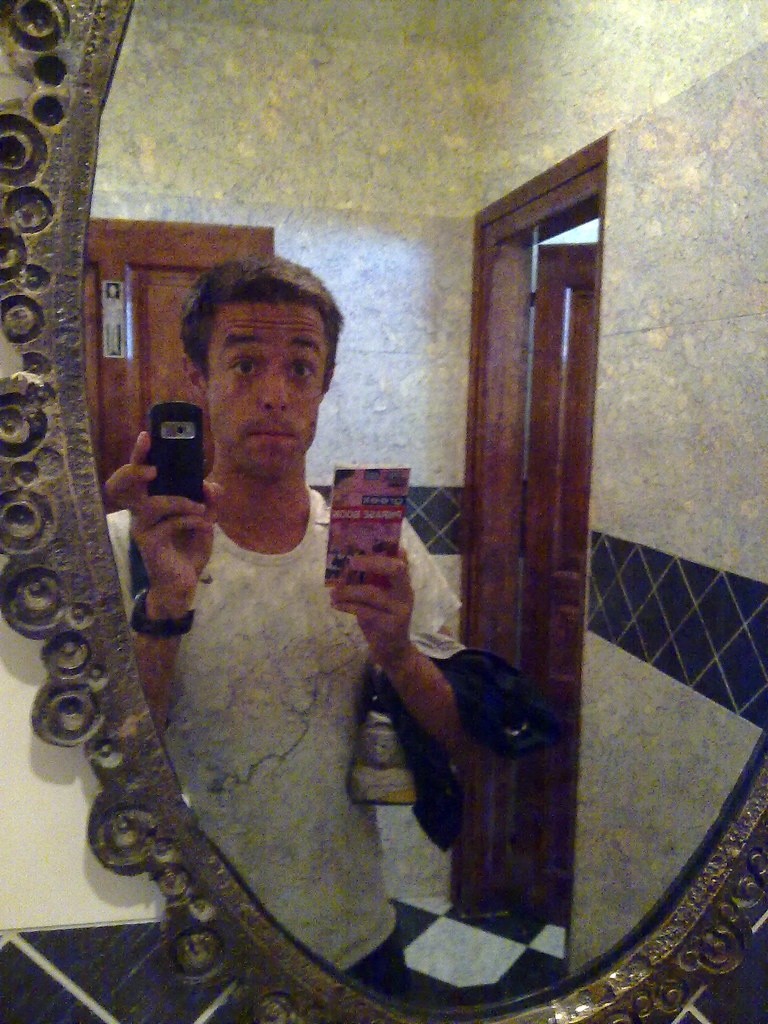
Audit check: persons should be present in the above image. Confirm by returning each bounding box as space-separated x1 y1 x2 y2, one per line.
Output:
105 252 468 1003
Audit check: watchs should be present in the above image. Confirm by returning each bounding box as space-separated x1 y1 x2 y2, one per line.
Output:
130 588 197 640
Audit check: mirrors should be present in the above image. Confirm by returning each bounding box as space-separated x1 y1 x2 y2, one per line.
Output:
0 0 768 1024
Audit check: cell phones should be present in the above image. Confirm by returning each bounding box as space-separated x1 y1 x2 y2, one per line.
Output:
146 400 203 505
